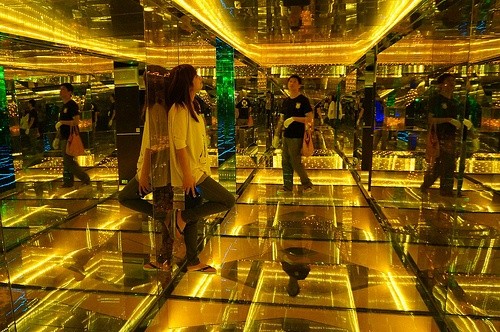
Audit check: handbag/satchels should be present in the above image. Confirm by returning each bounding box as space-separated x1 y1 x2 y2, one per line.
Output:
426 118 438 159
247 108 253 127
67 120 84 156
302 117 315 157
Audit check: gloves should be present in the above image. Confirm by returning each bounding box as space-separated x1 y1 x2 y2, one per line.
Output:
52 139 59 149
56 122 62 130
284 117 295 128
447 118 460 127
25 129 30 134
273 137 280 148
108 120 113 126
464 119 473 132
320 118 324 124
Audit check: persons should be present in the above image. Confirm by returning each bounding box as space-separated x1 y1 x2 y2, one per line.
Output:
236 90 337 130
276 222 472 314
273 75 314 193
21 65 170 272
167 63 236 275
339 73 482 200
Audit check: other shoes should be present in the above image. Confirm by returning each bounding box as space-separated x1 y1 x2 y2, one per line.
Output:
278 187 292 194
81 181 90 187
61 183 74 189
303 183 314 193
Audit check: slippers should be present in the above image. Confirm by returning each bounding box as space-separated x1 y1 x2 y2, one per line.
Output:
188 265 217 274
143 263 171 271
165 208 173 239
173 208 185 239
